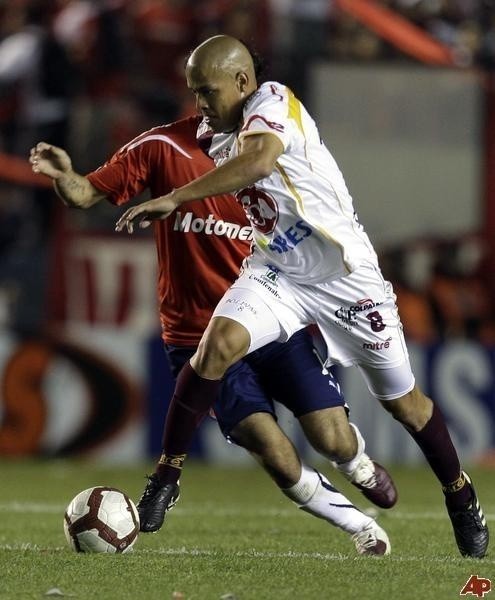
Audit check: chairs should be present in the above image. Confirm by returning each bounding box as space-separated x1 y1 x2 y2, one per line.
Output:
442 470 488 558
136 463 182 532
350 519 391 555
339 456 397 508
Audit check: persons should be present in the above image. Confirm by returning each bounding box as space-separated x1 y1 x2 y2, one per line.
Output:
0 0 495 342
114 35 488 559
30 116 397 557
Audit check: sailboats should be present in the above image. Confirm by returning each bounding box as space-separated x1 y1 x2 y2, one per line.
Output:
63 485 140 554
